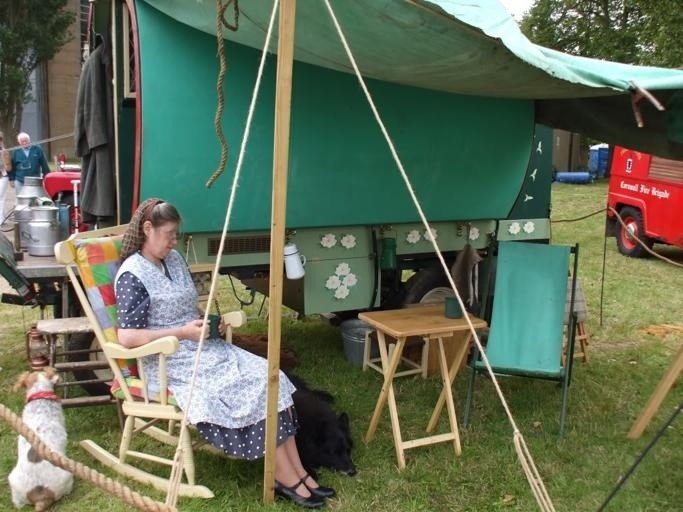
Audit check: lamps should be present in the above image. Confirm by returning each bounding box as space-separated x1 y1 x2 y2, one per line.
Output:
463 239 579 443
53 221 247 500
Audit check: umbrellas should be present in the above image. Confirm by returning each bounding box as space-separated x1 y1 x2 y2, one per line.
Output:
274 477 326 509
300 474 336 499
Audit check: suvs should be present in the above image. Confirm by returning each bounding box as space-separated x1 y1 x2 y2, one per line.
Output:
28 204 61 258
14 175 54 252
339 318 379 369
377 226 397 272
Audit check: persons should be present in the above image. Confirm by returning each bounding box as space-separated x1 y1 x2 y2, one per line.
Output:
112 197 337 509
0 131 12 179
7 132 50 194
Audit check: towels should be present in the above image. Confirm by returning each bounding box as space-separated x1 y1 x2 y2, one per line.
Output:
590 144 609 176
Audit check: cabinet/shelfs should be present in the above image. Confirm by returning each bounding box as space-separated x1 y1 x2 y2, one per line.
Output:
379 236 398 271
284 242 308 281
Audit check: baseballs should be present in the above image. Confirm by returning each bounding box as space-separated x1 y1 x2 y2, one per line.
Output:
59 152 65 168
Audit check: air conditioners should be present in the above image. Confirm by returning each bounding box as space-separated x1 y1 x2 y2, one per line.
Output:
7 366 75 512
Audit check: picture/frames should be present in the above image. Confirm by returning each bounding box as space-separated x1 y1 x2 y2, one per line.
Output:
355 302 487 471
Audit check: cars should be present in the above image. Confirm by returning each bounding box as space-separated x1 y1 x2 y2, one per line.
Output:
445 296 465 319
197 313 221 341
